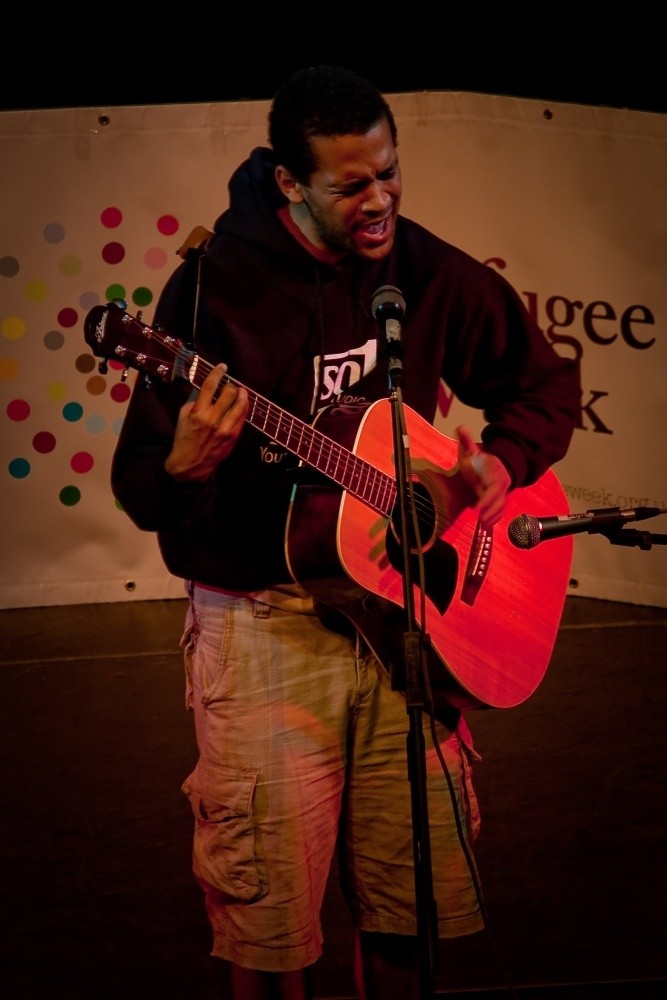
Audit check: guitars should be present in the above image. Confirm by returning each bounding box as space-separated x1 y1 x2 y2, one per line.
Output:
82 297 577 721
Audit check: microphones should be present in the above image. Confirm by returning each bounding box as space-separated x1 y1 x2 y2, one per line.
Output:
371 285 406 387
508 506 662 550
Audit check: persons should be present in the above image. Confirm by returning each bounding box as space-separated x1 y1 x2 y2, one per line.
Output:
111 64 580 1000
